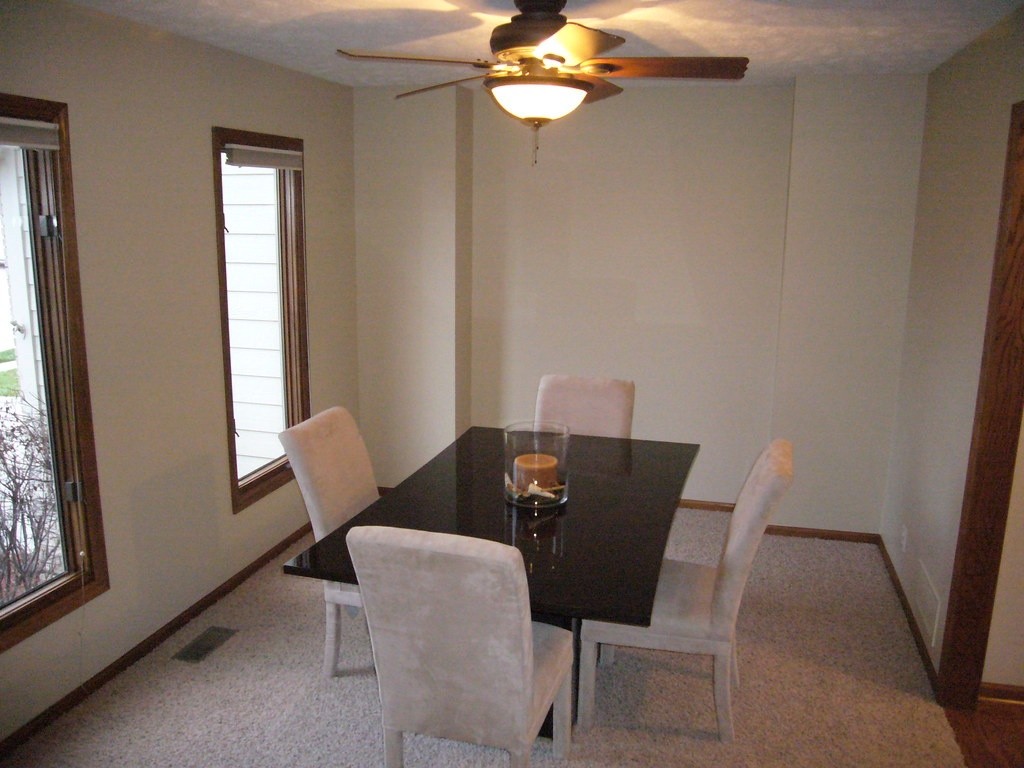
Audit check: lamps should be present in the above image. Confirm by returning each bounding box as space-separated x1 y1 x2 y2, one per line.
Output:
479 76 595 127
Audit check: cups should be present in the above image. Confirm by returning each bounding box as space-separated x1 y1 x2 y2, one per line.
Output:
503 421 570 508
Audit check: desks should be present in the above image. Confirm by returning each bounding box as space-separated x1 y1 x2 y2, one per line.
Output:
281 425 702 740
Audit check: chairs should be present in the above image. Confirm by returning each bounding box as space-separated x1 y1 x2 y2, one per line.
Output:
278 406 381 679
534 373 635 439
345 524 575 768
576 438 795 747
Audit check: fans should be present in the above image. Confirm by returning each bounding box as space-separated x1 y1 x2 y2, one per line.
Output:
337 0 749 108
